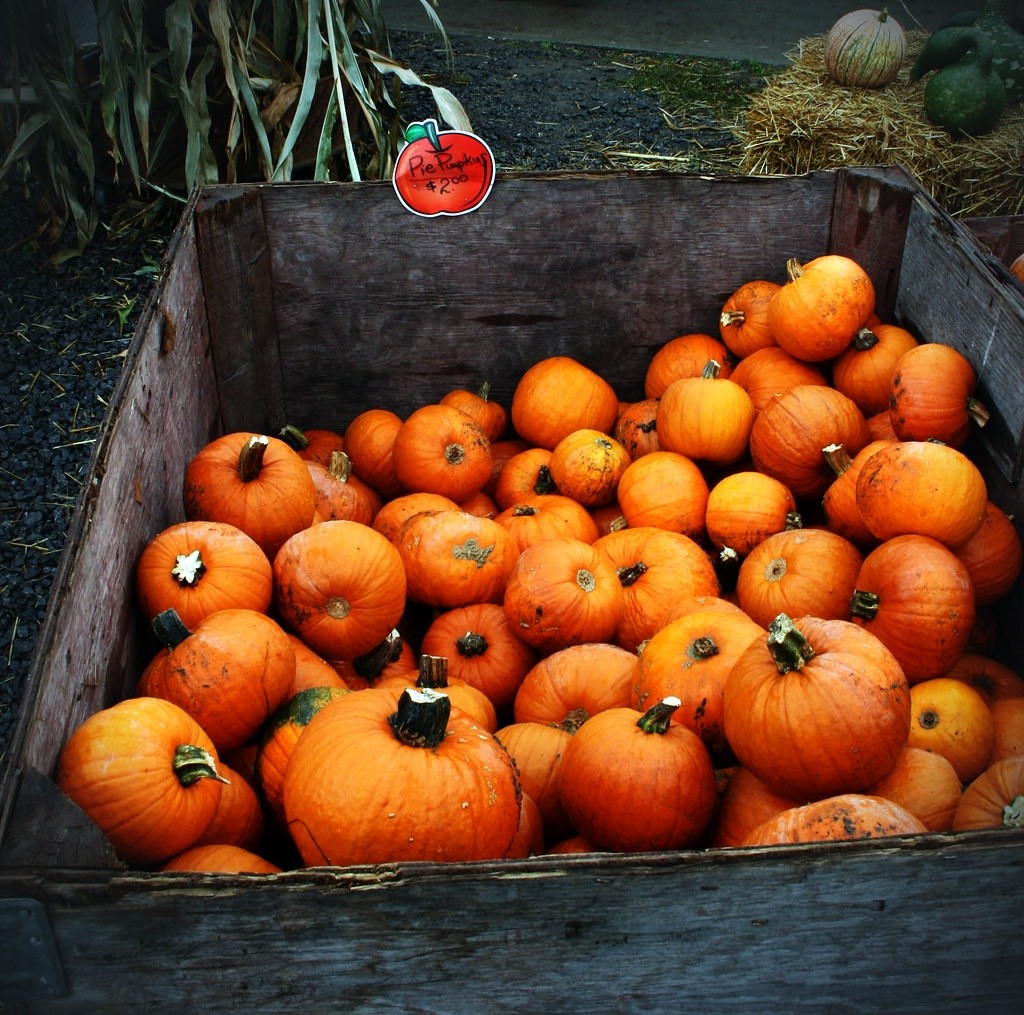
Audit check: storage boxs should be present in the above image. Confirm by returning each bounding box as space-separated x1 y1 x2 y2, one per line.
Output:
0 164 1024 1015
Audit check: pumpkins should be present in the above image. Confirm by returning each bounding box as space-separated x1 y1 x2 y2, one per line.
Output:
55 256 1024 873
824 7 905 89
